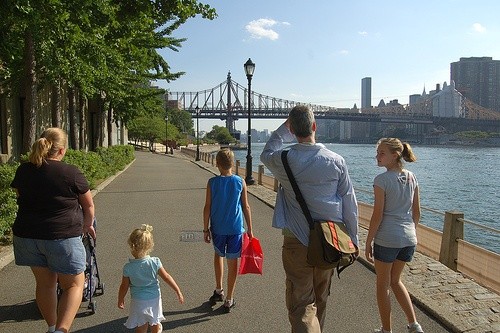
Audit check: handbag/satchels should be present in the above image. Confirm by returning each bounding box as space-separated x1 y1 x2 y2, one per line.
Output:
237 232 263 275
280 150 356 270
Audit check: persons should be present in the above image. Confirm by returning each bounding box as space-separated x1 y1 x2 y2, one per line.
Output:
260 105 359 333
203 149 253 313
365 137 422 333
118 223 183 333
10 128 96 333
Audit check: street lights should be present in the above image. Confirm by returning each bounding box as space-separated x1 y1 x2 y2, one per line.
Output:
195 106 200 161
164 114 169 154
244 58 256 186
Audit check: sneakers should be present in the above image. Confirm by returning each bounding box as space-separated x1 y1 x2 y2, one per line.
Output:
209 290 224 302
374 326 392 333
406 325 424 333
224 299 236 312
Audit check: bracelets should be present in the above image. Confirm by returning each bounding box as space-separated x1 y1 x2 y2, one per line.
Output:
203 229 209 233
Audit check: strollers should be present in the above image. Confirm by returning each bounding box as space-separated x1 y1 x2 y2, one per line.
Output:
56 205 105 314
